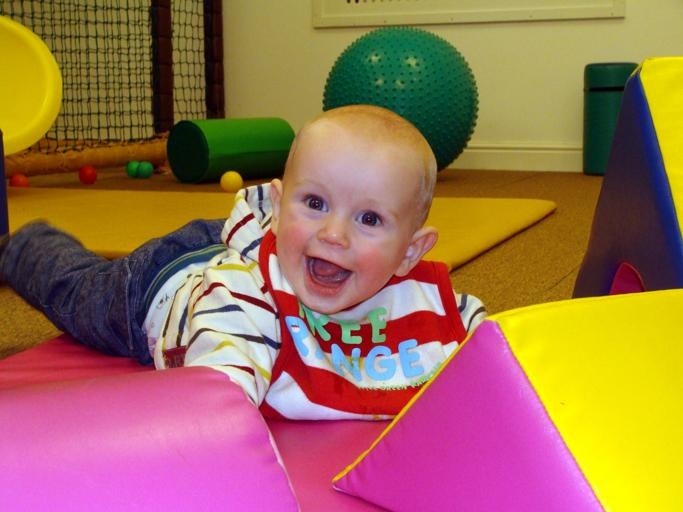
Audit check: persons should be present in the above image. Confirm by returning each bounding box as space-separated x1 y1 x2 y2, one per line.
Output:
0 104 486 424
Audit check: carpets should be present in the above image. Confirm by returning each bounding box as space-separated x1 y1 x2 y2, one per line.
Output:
2 181 556 272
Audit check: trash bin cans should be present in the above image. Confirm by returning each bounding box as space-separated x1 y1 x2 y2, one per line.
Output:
583 63 639 175
